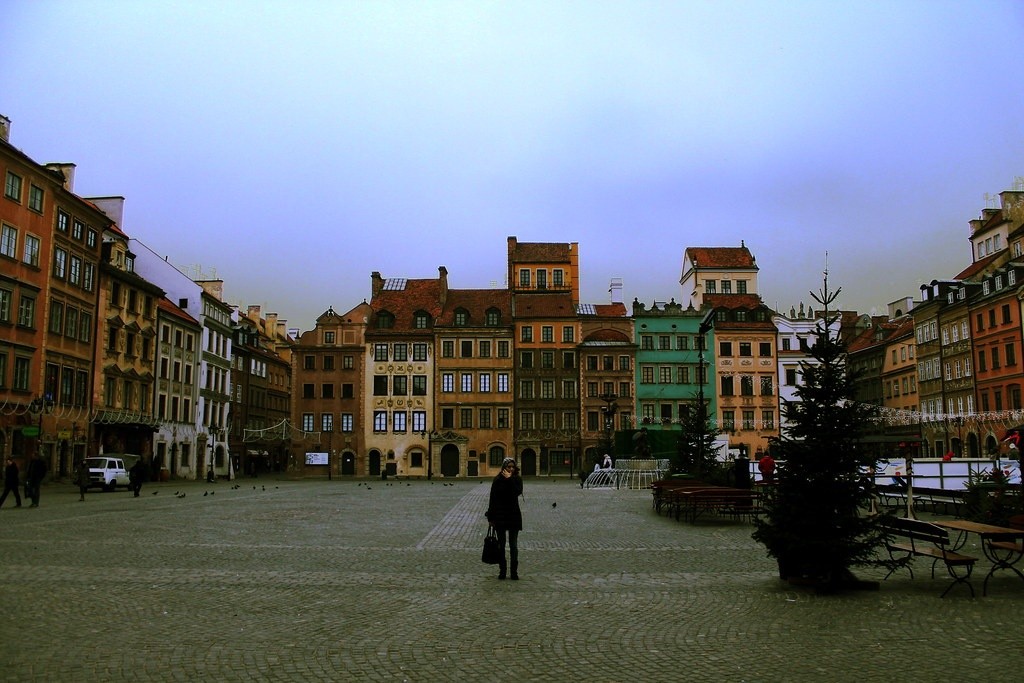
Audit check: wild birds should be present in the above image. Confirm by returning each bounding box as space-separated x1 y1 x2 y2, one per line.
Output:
276 487 278 489
432 482 433 484
231 484 240 489
443 483 454 486
151 491 186 499
386 483 393 486
358 482 372 490
204 490 208 496
253 485 265 491
480 481 482 484
552 502 556 508
553 479 555 482
211 491 215 495
407 484 410 486
400 481 401 484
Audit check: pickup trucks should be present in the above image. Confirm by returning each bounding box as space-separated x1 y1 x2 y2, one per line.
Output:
85 457 138 492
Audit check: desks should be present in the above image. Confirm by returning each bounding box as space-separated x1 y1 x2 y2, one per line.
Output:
932 521 1024 596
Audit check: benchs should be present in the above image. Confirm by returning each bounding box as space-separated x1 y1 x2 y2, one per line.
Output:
877 485 968 519
985 541 1024 581
650 480 770 523
878 515 979 599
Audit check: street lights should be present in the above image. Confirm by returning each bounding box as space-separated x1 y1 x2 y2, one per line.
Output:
30 391 55 451
208 424 221 483
563 426 582 479
321 424 338 479
600 389 618 455
953 417 965 458
697 308 718 479
421 428 439 481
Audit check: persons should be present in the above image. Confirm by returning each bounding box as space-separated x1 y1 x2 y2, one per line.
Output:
632 427 656 459
758 451 776 500
74 460 90 501
604 454 612 470
25 451 48 508
486 459 524 582
128 461 143 498
0 459 22 510
594 464 600 472
152 455 161 481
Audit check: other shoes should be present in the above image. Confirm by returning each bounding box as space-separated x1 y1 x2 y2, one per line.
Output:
510 569 519 580
498 569 506 580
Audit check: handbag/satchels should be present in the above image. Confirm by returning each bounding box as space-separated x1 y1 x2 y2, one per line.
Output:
482 525 502 564
25 481 30 500
